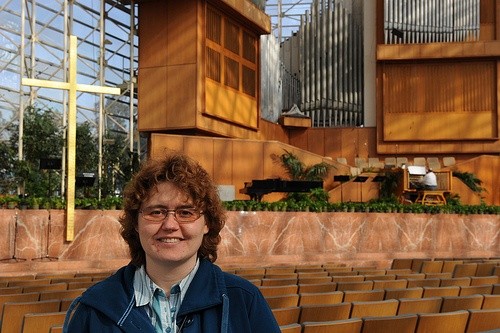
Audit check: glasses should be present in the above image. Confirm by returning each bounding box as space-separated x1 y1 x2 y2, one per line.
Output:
137 206 206 221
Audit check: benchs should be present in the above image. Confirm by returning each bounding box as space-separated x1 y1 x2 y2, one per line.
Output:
416 190 447 206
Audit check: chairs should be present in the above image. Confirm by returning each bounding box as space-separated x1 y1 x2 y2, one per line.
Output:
0 258 500 333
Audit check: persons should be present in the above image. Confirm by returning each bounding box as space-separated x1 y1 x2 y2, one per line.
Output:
62 149 280 333
417 167 438 191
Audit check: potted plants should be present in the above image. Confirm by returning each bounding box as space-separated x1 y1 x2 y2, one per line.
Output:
0 196 66 210
222 200 500 214
75 196 127 210
